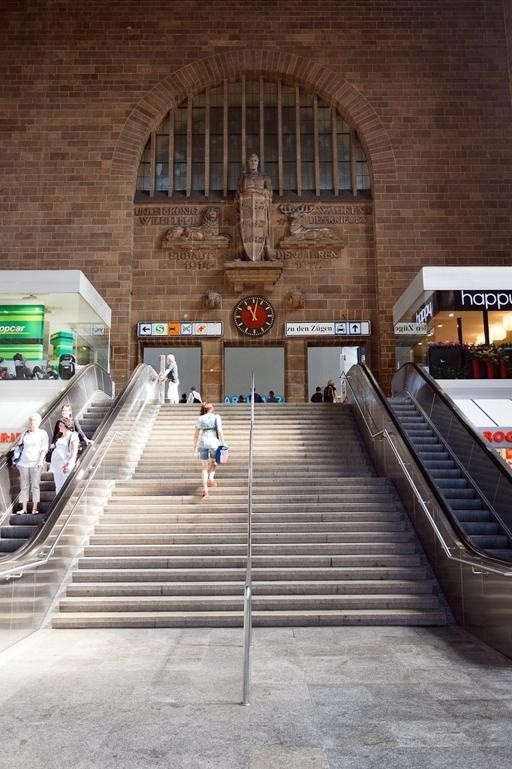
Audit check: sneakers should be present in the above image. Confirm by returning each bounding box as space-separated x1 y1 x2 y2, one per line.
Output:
200 490 207 498
208 481 217 487
17 510 27 515
32 509 39 515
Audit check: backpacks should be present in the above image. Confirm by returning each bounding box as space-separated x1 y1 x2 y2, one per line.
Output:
193 398 201 403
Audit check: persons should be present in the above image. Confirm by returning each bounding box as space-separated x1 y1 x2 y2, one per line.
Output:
179 386 202 403
239 388 278 403
193 402 229 497
237 153 273 262
0 352 58 380
11 413 49 515
310 379 336 403
49 404 95 496
160 354 179 404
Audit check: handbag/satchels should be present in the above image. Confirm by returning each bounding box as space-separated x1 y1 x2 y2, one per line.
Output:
46 449 54 462
168 371 175 380
216 446 228 464
6 450 21 468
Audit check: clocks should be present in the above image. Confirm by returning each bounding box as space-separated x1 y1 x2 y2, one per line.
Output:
233 295 275 336
